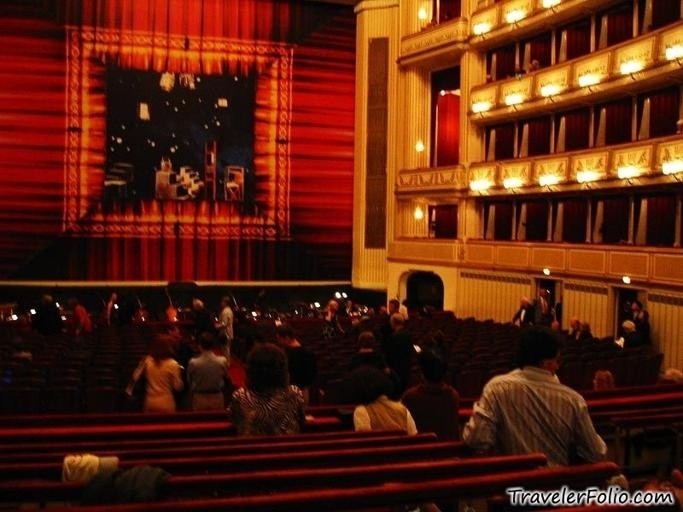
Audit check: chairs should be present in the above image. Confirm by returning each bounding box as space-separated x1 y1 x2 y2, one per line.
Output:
0 303 683 511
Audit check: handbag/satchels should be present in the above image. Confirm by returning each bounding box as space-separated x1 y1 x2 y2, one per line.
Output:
120 357 149 408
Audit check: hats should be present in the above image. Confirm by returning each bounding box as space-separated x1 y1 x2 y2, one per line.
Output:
61 451 122 488
